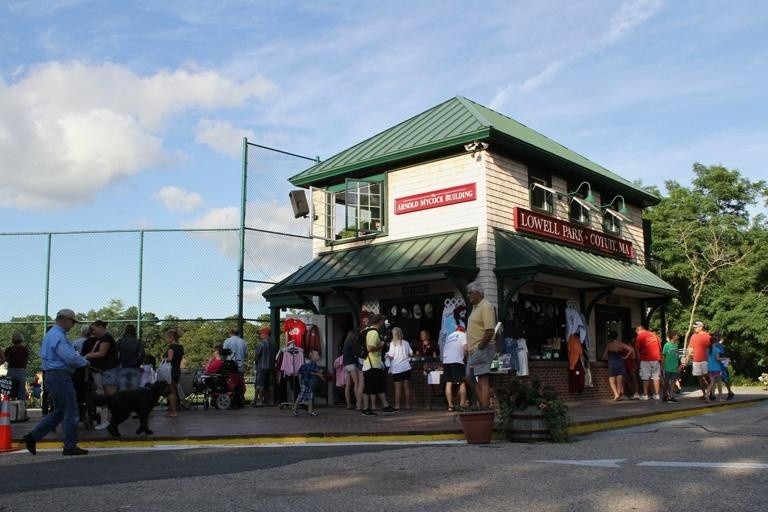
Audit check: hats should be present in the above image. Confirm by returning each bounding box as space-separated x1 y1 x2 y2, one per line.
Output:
259 329 272 335
56 309 81 325
692 321 704 327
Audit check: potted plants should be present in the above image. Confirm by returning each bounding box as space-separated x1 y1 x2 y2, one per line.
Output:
456 400 497 443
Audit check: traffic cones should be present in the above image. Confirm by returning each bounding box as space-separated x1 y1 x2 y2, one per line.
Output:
0 393 21 453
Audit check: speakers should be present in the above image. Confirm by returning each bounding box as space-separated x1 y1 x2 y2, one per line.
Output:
289 190 309 218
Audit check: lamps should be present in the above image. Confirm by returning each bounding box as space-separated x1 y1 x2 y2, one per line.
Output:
596 195 629 214
545 180 596 207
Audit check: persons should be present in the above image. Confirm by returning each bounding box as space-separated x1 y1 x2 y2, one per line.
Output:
602 321 735 403
22 309 91 455
333 311 413 417
202 326 249 409
375 221 382 231
291 351 326 416
1 326 53 416
443 283 496 412
251 326 272 407
73 320 184 439
416 330 437 357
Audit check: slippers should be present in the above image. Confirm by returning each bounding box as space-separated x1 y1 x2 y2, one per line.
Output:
164 407 177 417
250 398 276 408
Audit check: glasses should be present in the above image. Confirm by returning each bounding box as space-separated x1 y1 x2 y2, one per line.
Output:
467 291 473 295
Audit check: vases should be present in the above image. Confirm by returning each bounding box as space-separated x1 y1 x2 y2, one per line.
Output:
510 410 554 442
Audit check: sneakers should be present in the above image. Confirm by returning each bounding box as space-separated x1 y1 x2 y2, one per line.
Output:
292 408 319 417
698 389 735 402
346 402 412 417
614 390 680 402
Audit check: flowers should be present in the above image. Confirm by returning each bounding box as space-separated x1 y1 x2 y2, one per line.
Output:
493 376 578 442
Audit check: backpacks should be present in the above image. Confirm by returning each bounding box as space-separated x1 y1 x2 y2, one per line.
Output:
351 328 380 359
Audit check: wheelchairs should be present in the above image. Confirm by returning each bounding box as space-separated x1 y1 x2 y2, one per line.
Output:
201 354 248 411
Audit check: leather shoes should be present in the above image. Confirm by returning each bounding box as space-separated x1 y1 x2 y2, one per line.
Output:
22 432 90 455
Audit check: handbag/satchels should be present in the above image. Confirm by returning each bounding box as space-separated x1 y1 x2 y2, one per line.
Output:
156 360 172 385
79 365 97 389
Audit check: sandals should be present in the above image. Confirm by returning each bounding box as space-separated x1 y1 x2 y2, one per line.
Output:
447 404 469 412
